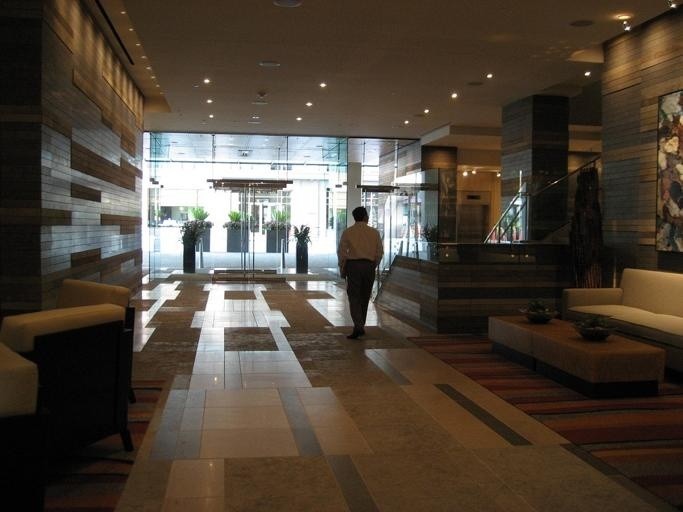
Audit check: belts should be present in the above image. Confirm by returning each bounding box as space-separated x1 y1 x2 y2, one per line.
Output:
348 259 369 262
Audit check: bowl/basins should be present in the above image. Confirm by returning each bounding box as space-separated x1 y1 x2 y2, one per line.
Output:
570 325 618 341
517 306 562 323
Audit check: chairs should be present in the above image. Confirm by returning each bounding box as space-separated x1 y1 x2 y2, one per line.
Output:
0 278 138 512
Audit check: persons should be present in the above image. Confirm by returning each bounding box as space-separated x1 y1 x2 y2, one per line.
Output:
337 204 384 340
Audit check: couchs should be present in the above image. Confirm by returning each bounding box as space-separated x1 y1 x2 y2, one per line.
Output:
561 267 683 374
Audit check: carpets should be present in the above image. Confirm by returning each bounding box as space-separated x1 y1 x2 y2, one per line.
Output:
407 335 683 512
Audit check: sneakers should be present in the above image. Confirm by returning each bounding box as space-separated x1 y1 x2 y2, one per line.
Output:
346 327 366 339
